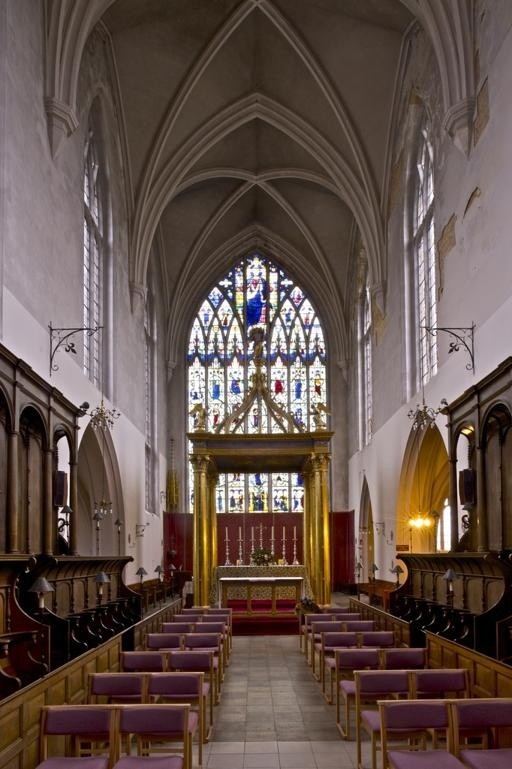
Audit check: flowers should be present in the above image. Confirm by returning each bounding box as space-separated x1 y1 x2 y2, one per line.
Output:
248 546 275 567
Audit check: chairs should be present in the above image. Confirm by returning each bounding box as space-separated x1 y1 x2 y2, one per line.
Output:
299 611 512 768
35 608 234 769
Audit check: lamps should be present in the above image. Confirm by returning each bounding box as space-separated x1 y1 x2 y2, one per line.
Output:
28 577 55 677
48 321 122 431
92 431 115 515
61 506 123 557
92 571 112 648
406 321 479 431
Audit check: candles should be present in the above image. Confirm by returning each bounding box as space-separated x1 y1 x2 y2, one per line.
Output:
224 526 297 540
